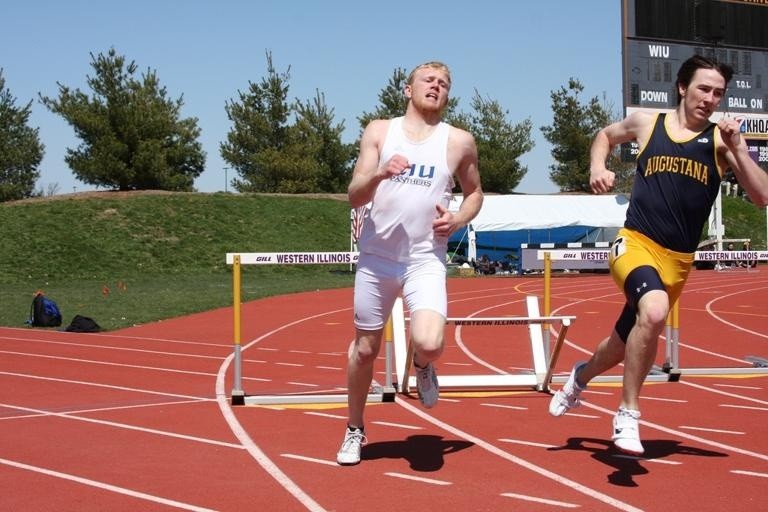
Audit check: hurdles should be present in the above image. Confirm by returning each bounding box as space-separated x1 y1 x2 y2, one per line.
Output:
537 249 681 382
663 249 768 374
391 252 578 393
226 251 396 406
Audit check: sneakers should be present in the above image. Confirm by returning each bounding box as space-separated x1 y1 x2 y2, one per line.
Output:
413 354 439 409
337 425 368 465
548 361 590 416
612 407 645 457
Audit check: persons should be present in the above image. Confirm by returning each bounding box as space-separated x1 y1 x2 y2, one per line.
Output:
546 53 767 454
740 243 757 268
692 238 717 270
724 243 740 268
336 61 485 466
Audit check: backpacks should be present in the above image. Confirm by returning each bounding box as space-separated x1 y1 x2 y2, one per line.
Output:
24 291 62 327
